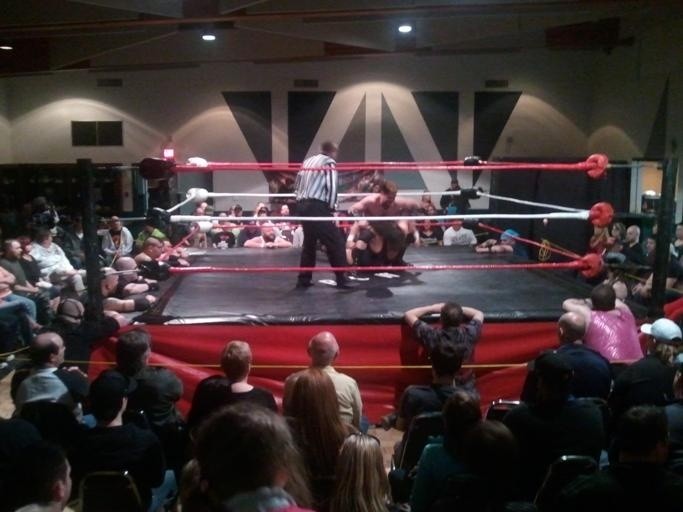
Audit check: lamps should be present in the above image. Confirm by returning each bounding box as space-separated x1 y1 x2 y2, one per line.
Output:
199 22 217 41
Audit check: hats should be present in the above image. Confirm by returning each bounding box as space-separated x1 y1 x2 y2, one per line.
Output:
640 317 682 342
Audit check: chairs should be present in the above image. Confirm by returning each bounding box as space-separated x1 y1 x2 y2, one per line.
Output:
10 378 149 512
395 377 683 512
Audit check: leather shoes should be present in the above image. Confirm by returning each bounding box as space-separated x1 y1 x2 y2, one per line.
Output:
335 280 362 290
296 279 315 287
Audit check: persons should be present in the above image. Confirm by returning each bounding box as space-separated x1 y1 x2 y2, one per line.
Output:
290 140 363 291
0 178 683 511
